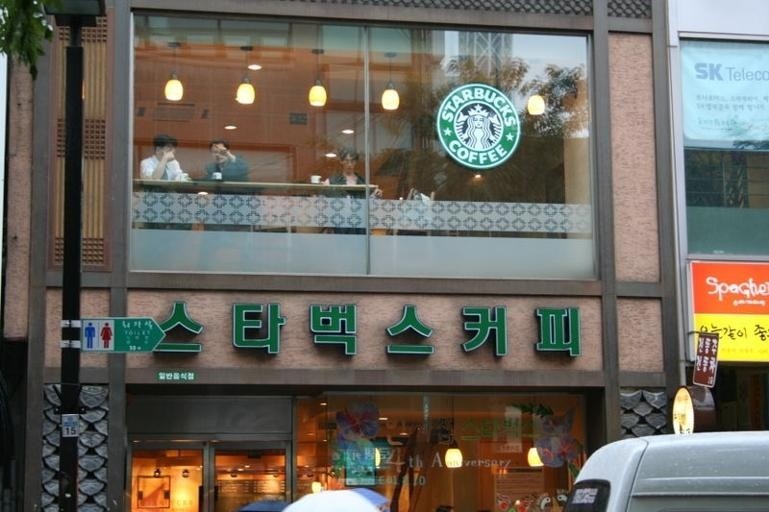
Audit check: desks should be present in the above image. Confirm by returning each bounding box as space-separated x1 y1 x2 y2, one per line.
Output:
132 178 378 270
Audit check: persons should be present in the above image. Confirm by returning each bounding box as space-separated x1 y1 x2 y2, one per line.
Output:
434 504 456 512
200 139 250 232
139 135 194 229
322 141 383 234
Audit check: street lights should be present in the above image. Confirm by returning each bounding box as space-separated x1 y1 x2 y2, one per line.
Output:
44 0 106 512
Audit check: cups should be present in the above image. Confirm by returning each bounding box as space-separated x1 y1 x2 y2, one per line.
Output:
180 173 189 182
311 175 321 185
211 171 222 180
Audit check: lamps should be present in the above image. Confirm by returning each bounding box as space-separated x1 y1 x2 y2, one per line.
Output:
445 394 463 469
381 52 400 110
528 94 546 116
236 45 256 105
526 448 545 467
308 49 328 107
165 42 184 102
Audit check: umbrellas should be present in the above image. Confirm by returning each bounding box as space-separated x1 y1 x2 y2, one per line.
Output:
238 500 289 511
281 488 389 511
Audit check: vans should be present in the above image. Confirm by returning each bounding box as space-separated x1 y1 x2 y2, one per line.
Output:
563 429 769 511
